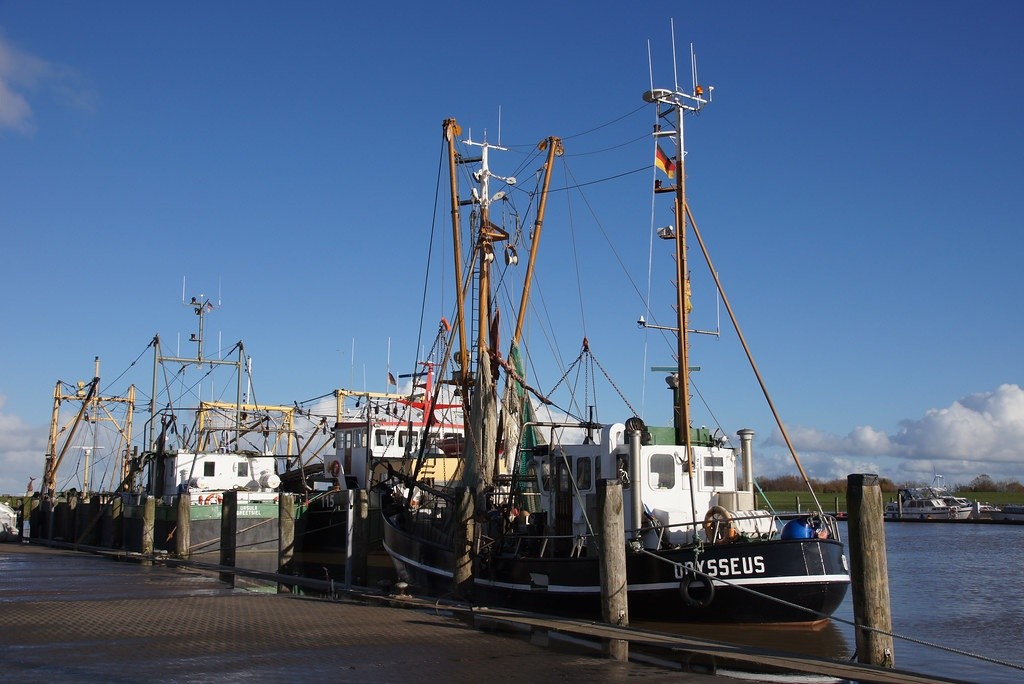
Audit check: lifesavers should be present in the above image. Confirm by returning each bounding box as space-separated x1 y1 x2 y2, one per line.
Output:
679 572 716 608
330 459 342 478
704 505 734 544
205 493 222 505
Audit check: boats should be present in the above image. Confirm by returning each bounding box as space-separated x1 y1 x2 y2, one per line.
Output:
897 486 973 508
988 503 1024 520
313 420 465 493
885 495 973 520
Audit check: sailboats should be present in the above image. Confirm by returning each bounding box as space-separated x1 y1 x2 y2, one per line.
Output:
17 277 310 575
381 18 847 631
